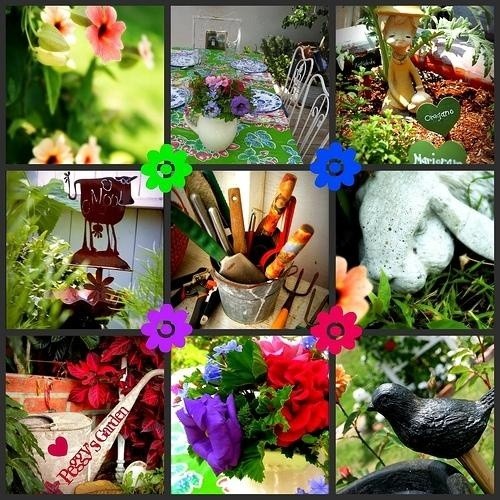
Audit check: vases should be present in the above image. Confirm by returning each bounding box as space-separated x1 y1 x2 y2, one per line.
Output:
196 111 239 153
225 448 324 494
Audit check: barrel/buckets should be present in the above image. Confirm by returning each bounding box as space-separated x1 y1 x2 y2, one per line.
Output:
210 231 287 325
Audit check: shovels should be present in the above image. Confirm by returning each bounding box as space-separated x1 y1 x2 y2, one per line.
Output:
170 203 269 285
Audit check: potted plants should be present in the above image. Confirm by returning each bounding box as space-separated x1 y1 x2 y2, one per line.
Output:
282 6 333 107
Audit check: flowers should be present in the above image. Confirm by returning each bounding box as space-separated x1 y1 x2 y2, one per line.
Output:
170 335 330 495
186 68 257 122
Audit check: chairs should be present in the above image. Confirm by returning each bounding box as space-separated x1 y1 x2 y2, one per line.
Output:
192 16 243 53
277 43 330 165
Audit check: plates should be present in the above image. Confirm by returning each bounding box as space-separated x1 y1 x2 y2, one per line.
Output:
171 85 191 109
231 59 267 73
251 89 282 112
171 50 201 67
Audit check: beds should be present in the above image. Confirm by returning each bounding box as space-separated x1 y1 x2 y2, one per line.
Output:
171 46 304 164
